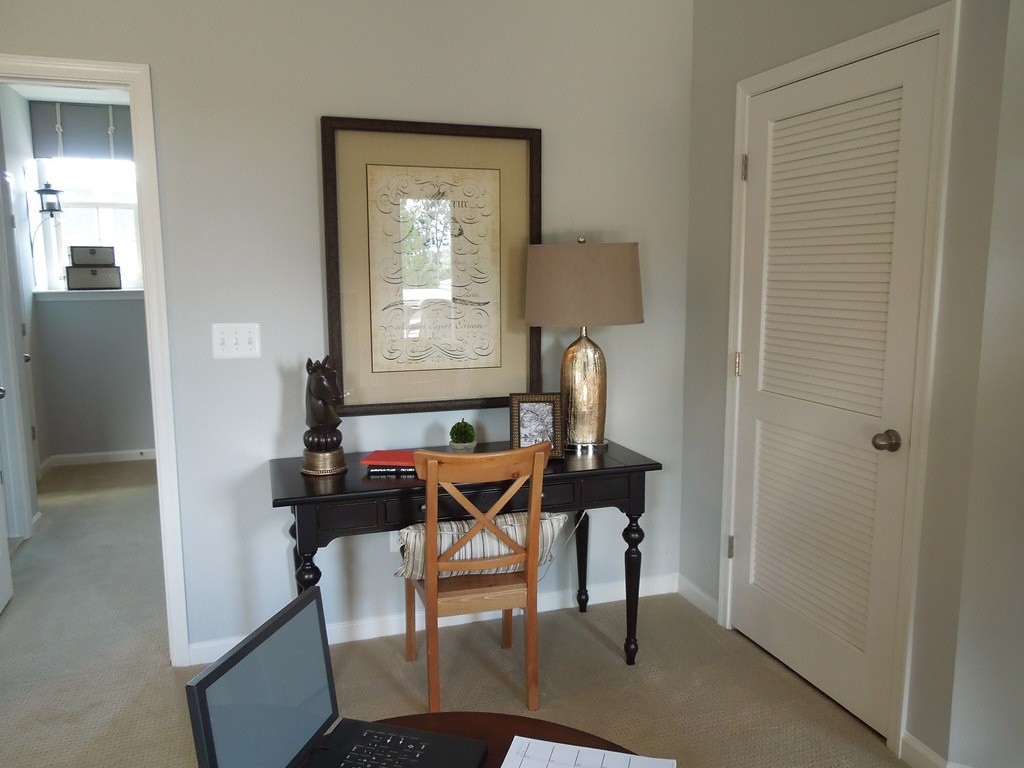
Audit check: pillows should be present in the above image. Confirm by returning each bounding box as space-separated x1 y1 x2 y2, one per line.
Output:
392 512 569 578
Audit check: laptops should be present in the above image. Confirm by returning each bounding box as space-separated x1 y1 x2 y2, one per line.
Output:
185 585 490 768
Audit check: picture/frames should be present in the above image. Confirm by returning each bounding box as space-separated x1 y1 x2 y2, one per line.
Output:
320 114 542 417
507 393 566 459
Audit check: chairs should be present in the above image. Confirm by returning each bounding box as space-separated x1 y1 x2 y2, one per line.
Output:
396 440 550 713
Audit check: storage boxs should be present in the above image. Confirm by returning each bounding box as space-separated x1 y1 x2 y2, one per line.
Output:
64 264 123 290
68 246 116 266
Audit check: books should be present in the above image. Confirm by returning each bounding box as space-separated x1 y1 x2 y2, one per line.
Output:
361 451 416 474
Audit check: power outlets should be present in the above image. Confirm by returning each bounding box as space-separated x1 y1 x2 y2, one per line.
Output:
391 531 404 551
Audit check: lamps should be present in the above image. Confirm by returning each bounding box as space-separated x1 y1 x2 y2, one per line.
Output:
32 182 65 253
526 237 645 452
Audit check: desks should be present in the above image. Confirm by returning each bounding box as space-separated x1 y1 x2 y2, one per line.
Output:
371 714 640 768
268 438 662 664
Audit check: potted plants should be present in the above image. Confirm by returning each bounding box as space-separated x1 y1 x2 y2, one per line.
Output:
449 418 477 453
